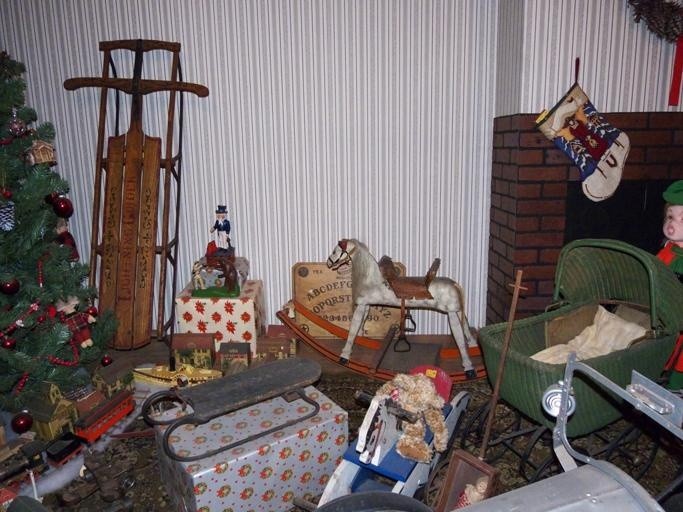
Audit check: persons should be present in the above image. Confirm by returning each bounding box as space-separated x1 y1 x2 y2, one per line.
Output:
652 179 683 390
210 204 231 252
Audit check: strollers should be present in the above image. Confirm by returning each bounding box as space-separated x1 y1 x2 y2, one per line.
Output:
461 240 683 504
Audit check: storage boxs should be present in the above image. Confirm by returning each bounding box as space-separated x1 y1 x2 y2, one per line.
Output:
174 280 266 357
152 385 349 512
